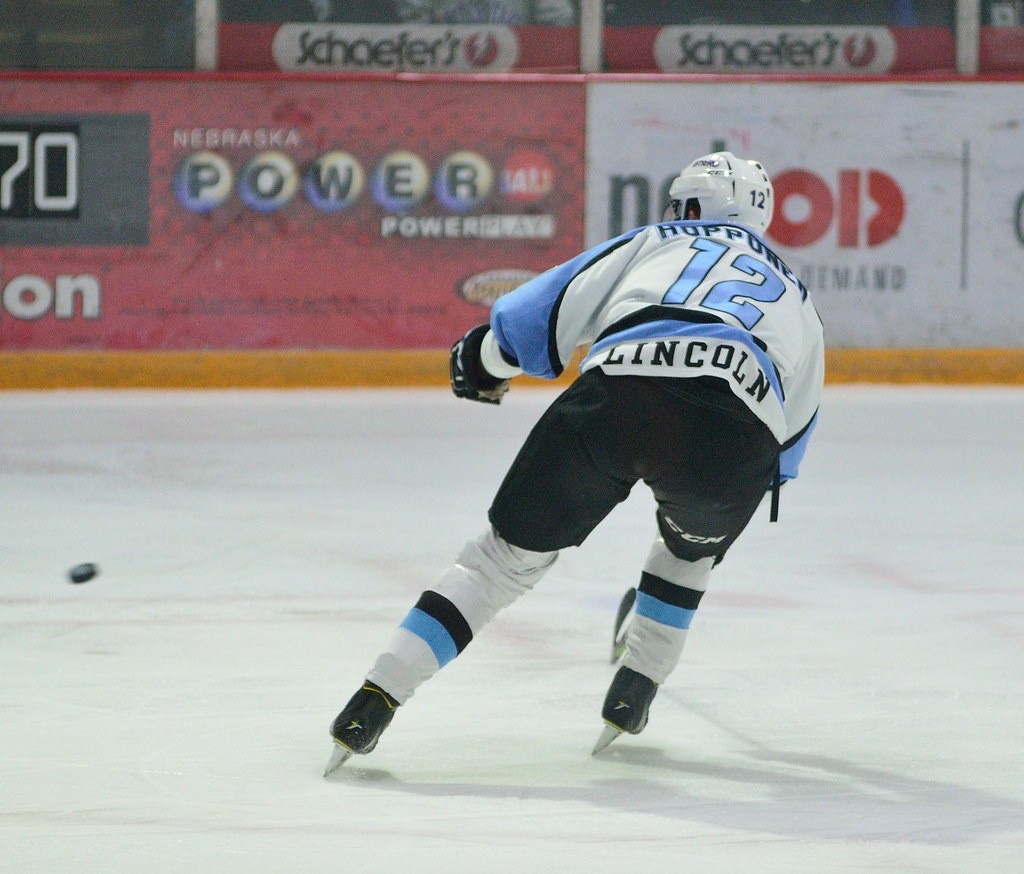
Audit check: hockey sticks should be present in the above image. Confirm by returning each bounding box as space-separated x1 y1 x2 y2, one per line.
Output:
610 584 640 664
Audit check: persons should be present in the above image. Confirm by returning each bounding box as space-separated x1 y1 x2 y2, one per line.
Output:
327 150 825 775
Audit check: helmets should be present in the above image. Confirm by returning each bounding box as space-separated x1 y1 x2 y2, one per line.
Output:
668 152 774 237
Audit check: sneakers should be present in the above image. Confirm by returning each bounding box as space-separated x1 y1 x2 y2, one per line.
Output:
323 680 399 777
592 666 658 755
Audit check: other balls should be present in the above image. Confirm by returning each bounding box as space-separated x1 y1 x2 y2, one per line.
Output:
70 562 98 584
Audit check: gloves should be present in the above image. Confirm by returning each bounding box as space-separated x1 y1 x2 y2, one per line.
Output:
448 323 511 405
